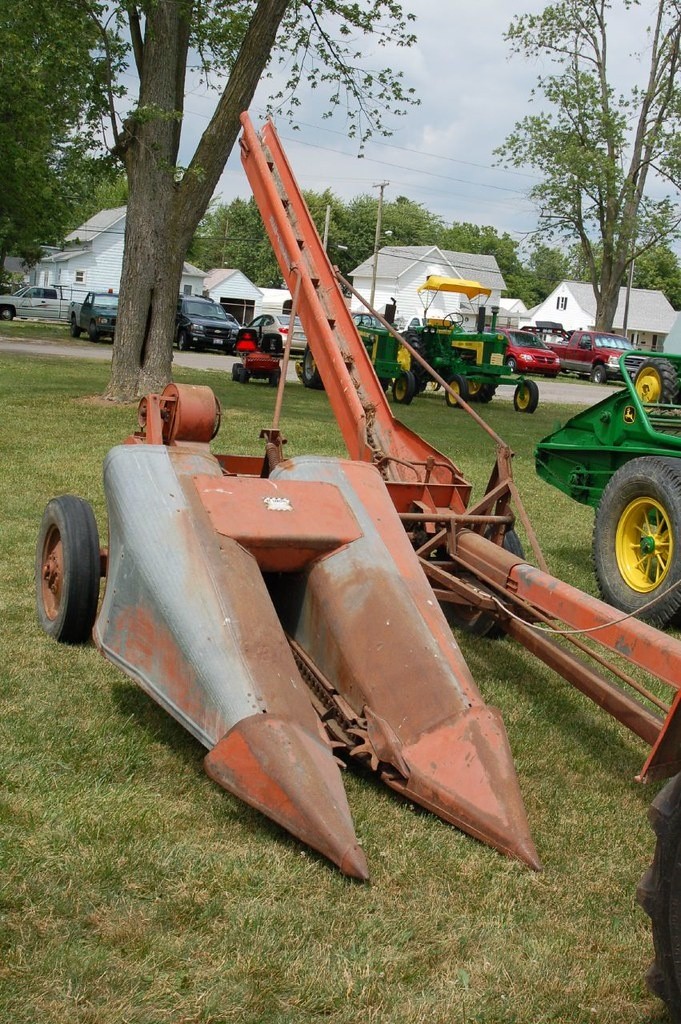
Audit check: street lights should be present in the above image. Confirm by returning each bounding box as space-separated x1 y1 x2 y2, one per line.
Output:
369 230 394 306
321 241 349 254
221 260 229 269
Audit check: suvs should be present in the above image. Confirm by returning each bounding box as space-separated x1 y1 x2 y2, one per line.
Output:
173 291 240 354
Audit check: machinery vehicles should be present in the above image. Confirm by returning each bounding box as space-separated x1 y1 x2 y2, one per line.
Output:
293 274 540 415
531 345 681 633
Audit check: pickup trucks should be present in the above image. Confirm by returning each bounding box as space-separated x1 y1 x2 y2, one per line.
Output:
519 326 637 386
67 288 119 342
0 286 71 322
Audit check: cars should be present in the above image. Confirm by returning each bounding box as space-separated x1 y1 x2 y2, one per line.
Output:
217 309 307 354
462 324 561 378
